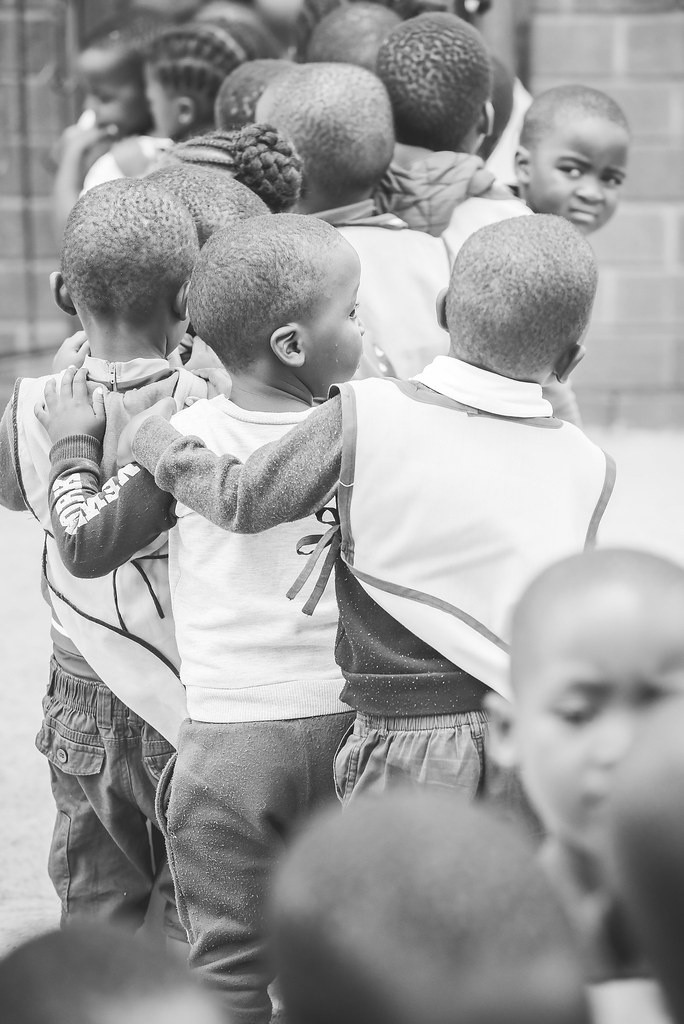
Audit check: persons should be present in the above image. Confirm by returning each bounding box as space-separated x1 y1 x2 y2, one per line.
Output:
37 209 389 1024
2 0 631 931
265 788 597 1024
0 926 226 1022
587 700 684 1024
112 212 615 798
485 546 684 984
